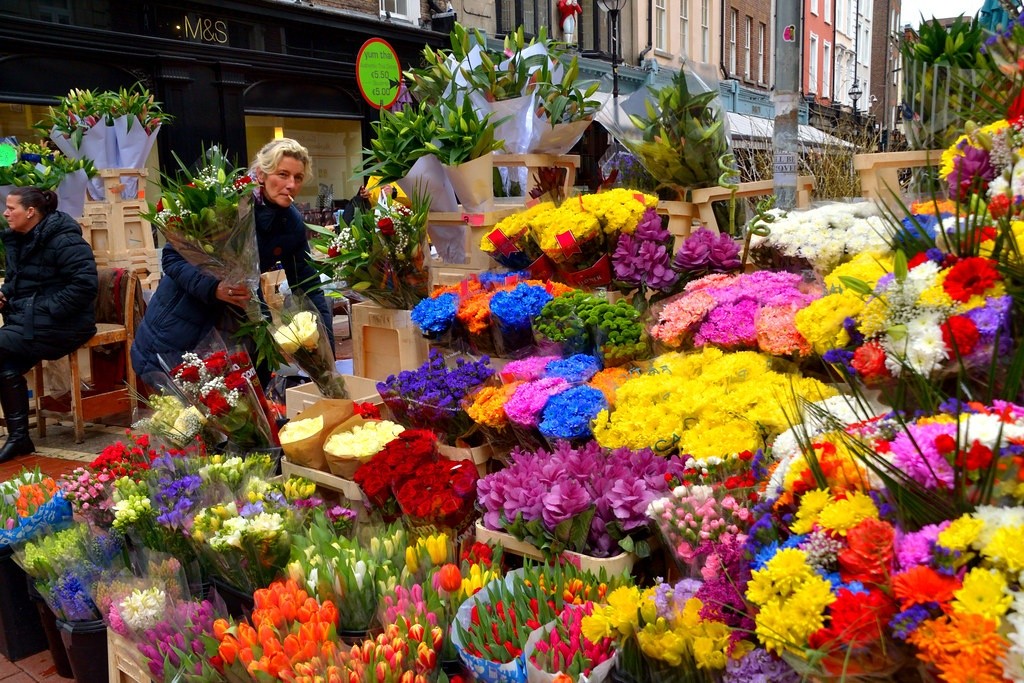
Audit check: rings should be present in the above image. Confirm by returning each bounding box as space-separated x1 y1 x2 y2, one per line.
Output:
228 290 232 296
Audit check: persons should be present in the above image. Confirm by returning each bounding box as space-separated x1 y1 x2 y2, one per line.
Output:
129 137 337 396
0 186 99 462
359 169 411 209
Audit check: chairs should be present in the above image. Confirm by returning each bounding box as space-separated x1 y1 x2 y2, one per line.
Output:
31 268 139 445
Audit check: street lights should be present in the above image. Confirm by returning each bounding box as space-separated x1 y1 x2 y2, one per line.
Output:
848 82 863 124
598 0 626 147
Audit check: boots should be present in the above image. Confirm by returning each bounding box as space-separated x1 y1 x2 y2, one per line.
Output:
0 371 35 464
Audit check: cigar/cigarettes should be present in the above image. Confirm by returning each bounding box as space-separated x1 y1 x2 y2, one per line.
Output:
289 195 294 201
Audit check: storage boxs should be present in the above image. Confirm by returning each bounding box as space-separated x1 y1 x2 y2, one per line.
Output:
286 374 383 419
349 300 429 380
106 627 154 683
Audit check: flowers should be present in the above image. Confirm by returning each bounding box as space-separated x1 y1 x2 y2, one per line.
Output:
0 8 1024 683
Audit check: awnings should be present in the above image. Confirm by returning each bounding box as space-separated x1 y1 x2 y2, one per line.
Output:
568 89 862 156
